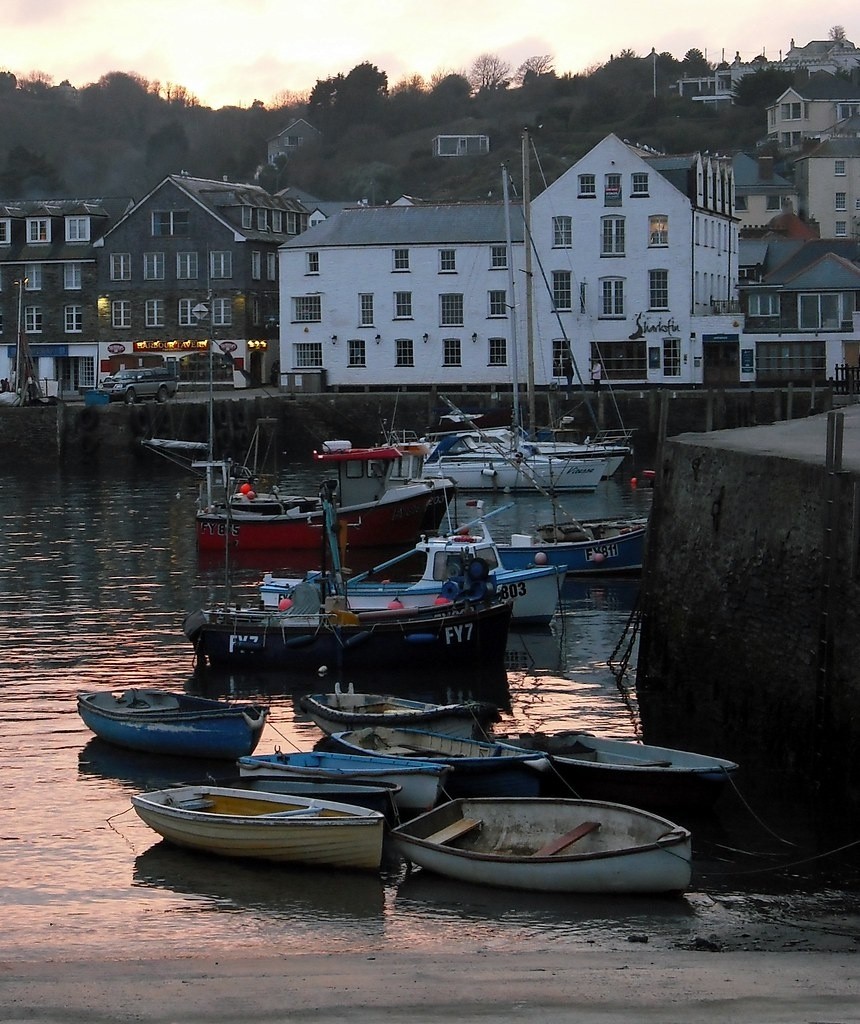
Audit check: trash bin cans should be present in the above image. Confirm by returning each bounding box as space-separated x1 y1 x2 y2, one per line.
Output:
279 367 325 393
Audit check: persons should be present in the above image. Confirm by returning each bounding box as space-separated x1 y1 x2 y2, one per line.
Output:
589 360 601 392
564 360 574 392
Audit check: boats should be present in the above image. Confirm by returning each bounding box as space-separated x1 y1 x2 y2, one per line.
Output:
235 749 456 813
170 770 402 817
300 682 501 744
329 728 553 794
387 797 695 894
134 125 648 674
132 784 386 873
76 684 273 760
491 731 741 801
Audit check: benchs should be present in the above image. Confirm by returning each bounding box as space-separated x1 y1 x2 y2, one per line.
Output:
373 745 430 754
259 808 324 817
179 799 214 810
531 821 601 856
423 817 483 845
619 760 672 767
110 706 180 713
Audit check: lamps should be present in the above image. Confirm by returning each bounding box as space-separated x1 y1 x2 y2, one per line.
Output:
422 333 428 343
331 336 337 344
472 333 477 342
374 335 381 344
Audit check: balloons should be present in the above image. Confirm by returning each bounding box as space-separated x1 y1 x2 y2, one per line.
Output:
388 600 404 609
435 598 449 605
279 599 293 611
241 484 255 499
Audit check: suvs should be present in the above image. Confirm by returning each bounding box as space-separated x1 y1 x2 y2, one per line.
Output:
95 367 178 404
